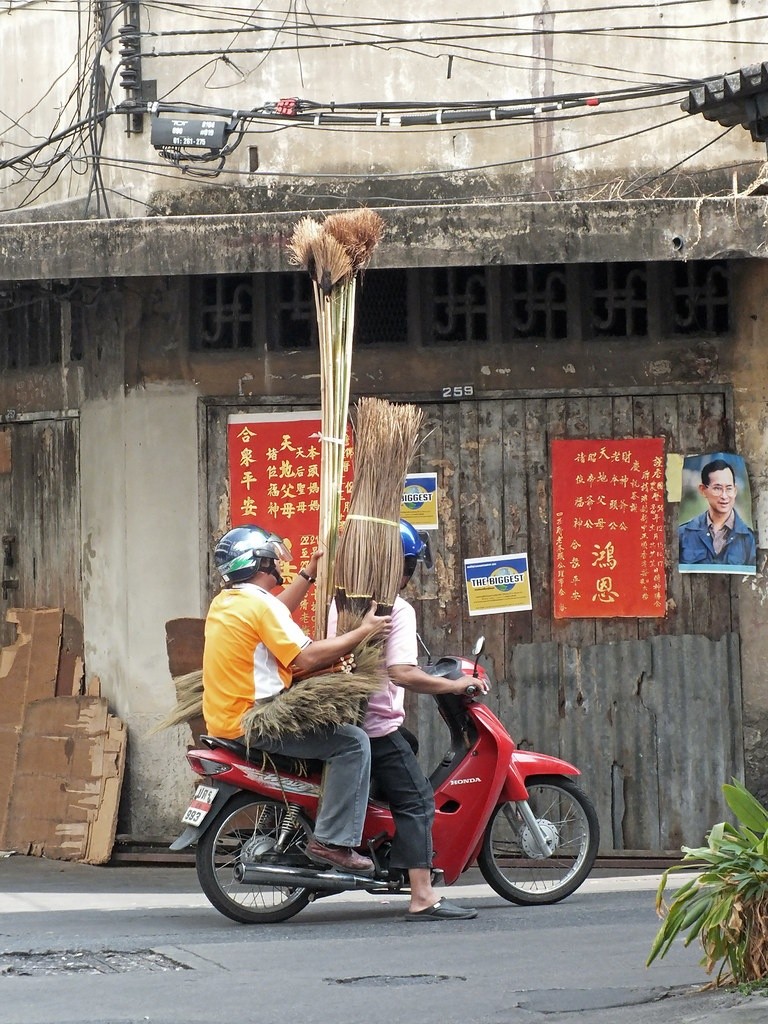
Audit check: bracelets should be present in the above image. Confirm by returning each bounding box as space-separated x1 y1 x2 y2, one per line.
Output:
297 568 316 584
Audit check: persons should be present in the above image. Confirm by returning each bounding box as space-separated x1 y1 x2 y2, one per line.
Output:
202 525 375 874
677 459 756 565
326 519 488 921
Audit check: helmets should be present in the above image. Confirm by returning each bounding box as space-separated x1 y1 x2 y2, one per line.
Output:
214 524 293 588
399 518 434 576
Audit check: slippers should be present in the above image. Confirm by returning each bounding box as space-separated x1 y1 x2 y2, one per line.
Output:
404 896 479 922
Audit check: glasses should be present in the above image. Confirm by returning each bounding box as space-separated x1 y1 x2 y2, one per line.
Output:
707 485 738 497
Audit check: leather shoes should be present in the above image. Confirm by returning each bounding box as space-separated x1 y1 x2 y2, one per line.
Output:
304 838 375 873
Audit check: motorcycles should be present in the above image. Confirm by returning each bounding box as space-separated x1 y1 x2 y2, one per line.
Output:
166 629 603 926
